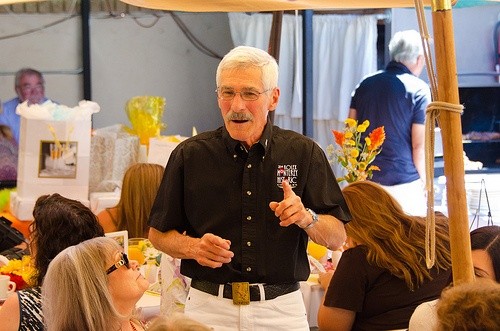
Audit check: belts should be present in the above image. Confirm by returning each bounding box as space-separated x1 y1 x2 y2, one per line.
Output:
190 277 300 306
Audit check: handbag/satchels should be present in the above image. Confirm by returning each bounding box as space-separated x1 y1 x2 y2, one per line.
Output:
0 216 25 254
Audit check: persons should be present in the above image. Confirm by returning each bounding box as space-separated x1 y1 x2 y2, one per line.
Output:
342 30 432 218
0 164 213 331
147 45 353 331
317 181 500 331
0 67 59 147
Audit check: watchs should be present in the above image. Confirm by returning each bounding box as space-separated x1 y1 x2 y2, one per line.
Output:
306 208 318 229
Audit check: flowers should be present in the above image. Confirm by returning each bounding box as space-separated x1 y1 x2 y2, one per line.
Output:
326 119 385 182
1 256 39 289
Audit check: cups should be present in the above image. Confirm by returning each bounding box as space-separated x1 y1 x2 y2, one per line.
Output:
125 238 147 265
308 238 328 274
0 275 16 298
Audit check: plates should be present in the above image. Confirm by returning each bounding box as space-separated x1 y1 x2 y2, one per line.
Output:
0 292 17 302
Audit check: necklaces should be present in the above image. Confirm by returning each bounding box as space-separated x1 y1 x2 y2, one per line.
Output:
129 320 138 331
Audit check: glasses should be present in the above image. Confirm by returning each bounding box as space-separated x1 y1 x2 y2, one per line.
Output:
215 85 273 102
106 252 130 275
